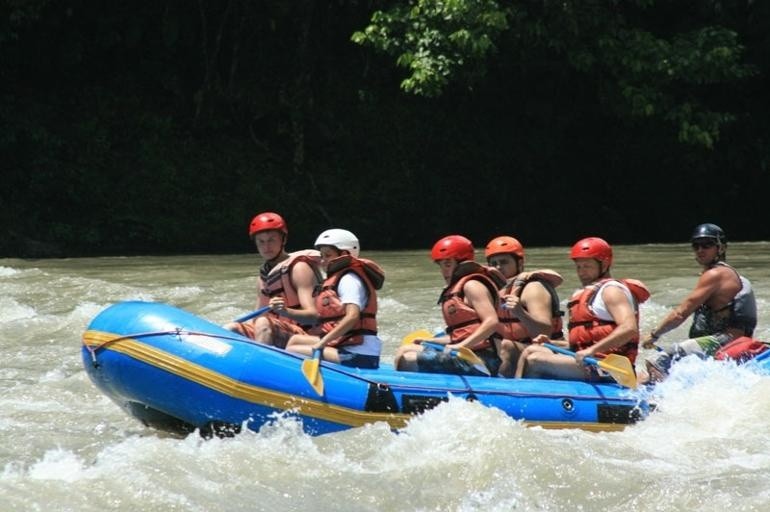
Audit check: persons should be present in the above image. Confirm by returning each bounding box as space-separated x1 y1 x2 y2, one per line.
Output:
285 228 381 368
224 213 322 348
515 237 637 385
393 234 503 377
485 236 564 378
643 223 757 385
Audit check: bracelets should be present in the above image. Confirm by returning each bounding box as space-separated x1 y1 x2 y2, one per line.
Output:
650 329 659 340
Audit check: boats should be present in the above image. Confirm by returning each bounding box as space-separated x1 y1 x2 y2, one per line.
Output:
82 302 770 436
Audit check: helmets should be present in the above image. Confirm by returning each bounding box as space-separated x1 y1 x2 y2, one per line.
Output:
485 236 524 261
315 229 359 258
249 214 288 237
691 224 727 246
431 236 474 263
571 238 612 268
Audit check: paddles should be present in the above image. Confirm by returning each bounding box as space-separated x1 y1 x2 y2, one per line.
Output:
403 327 446 345
423 340 486 366
539 343 637 389
302 349 324 397
